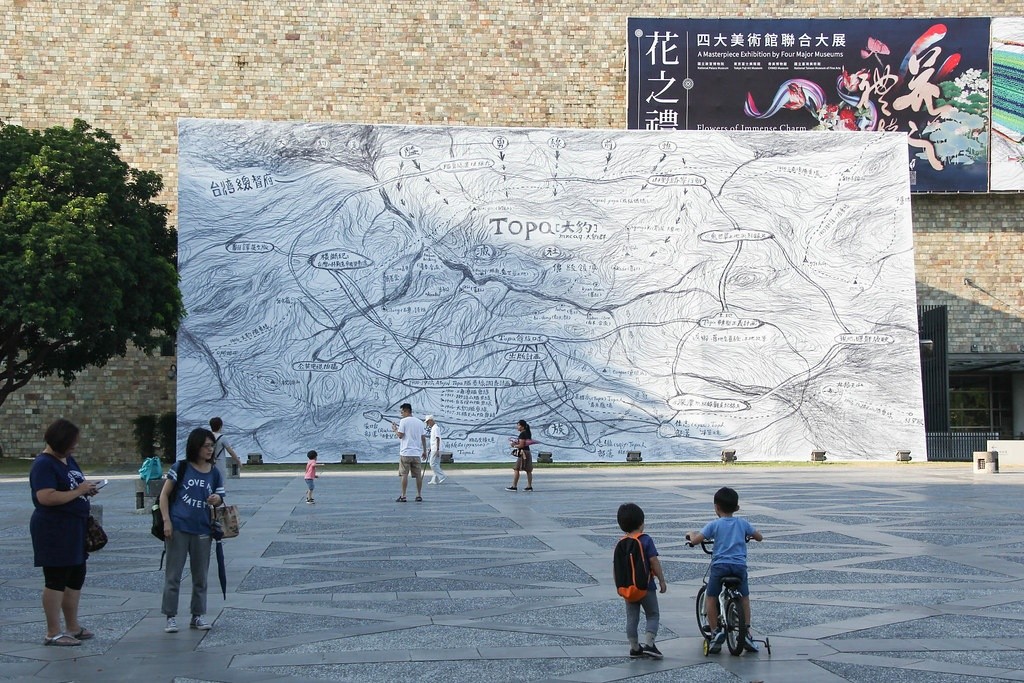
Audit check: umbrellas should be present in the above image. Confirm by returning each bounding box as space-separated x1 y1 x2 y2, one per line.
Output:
213 493 227 601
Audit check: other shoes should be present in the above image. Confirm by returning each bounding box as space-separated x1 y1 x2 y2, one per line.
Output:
306 498 315 504
439 477 448 483
428 480 438 485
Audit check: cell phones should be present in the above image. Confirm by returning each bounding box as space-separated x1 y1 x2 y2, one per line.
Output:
96 478 108 490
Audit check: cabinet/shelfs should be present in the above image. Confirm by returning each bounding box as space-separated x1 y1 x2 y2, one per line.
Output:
949 373 1013 432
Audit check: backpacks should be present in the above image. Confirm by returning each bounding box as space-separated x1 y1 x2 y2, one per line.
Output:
613 533 655 602
201 443 216 449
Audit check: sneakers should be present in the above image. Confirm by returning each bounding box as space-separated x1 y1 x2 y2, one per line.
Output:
190 615 212 629
522 487 532 491
165 617 179 633
642 643 662 657
708 627 726 653
630 644 649 657
505 486 517 492
736 631 759 652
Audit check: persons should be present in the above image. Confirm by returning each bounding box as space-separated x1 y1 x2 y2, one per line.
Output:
392 403 427 503
617 503 666 659
304 450 325 503
29 420 99 647
425 415 446 485
210 417 242 484
505 420 533 492
686 487 762 655
160 429 225 634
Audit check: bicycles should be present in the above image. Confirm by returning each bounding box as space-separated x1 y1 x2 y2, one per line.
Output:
683 534 773 657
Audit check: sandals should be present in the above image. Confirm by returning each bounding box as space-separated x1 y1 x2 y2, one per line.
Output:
396 496 406 502
44 633 82 646
415 496 422 502
74 627 95 639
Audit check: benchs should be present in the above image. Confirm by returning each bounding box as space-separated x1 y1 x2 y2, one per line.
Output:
135 479 166 514
225 457 240 478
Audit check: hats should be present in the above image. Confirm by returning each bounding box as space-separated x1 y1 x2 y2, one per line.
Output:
424 415 433 423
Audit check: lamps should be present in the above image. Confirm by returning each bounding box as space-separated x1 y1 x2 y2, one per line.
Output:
537 451 553 463
341 454 357 464
248 453 263 465
441 453 453 463
720 449 736 461
812 452 827 462
626 451 642 462
896 450 912 461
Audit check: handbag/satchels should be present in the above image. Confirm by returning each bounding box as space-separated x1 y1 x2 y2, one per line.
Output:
84 516 108 552
511 450 522 457
138 457 162 494
151 460 188 541
210 494 239 538
